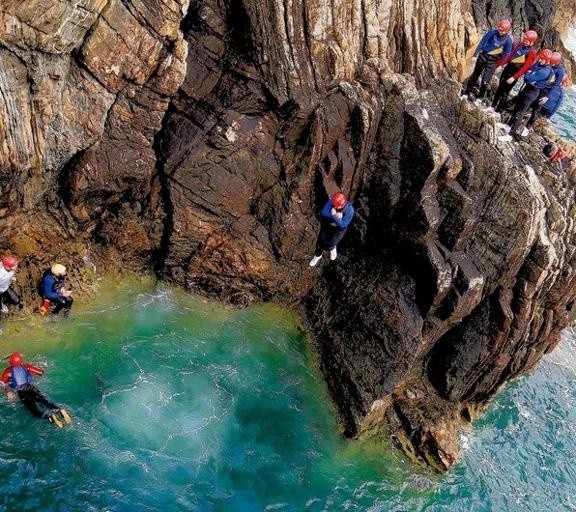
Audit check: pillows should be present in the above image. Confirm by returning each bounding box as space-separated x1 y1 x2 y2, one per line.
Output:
2 256 20 269
332 193 347 210
523 30 538 43
539 48 552 64
51 264 68 277
550 52 562 66
10 352 23 367
498 19 512 33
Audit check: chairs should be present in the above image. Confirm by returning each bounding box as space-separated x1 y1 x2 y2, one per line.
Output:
330 245 337 260
497 134 513 142
309 255 322 267
520 127 530 137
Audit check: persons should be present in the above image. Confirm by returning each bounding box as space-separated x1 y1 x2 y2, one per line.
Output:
466 18 570 138
44 263 74 312
0 256 22 314
309 193 354 268
0 352 71 428
